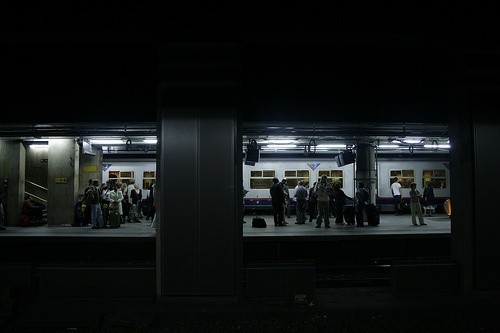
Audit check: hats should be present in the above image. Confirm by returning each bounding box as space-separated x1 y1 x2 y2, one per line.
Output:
411 182 417 186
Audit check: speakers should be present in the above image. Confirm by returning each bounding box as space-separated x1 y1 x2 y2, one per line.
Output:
335 152 355 167
244 150 259 166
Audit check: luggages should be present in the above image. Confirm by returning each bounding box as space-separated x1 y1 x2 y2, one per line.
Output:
344 200 356 225
109 202 120 229
367 203 380 226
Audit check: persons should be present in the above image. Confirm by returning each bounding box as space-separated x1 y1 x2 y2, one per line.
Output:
0 195 5 230
422 184 437 217
408 184 426 225
84 175 157 228
270 177 368 229
390 177 404 215
23 195 43 225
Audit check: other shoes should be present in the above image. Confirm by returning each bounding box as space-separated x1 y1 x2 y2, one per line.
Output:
92 225 108 229
274 216 345 228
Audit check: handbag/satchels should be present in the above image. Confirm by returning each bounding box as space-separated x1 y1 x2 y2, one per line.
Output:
85 186 94 203
